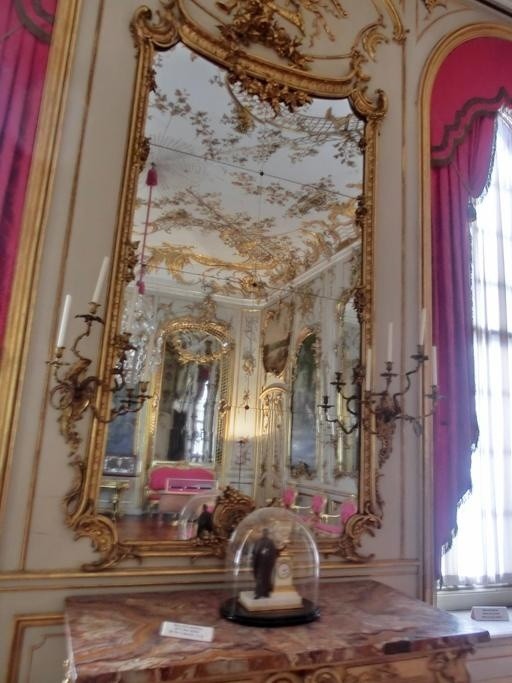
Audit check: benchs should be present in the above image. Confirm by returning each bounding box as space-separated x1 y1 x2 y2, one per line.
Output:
99 480 129 521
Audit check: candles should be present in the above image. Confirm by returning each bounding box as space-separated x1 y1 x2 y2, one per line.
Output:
366 347 371 389
127 284 141 333
388 321 394 361
58 294 73 346
432 346 438 384
91 255 111 302
129 340 151 391
419 306 427 345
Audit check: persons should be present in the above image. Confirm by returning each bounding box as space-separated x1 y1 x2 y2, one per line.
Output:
186 502 213 538
251 525 288 600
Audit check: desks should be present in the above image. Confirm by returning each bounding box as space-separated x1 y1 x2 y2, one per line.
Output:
64 580 512 681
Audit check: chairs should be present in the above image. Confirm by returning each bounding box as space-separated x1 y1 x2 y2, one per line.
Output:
262 485 358 540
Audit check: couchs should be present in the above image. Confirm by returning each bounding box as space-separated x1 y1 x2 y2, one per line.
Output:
143 460 219 517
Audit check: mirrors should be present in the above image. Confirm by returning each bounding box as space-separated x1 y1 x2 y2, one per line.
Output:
288 278 362 482
66 3 384 565
146 291 237 467
